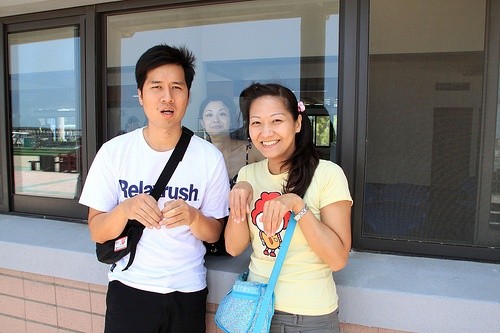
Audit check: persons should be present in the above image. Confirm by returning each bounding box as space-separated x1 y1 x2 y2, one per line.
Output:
198 93 267 189
225 83 353 333
78 42 231 333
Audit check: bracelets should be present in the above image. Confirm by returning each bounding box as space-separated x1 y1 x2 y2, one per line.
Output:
294 203 309 222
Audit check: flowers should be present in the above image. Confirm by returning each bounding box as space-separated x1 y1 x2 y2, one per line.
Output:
297 101 306 112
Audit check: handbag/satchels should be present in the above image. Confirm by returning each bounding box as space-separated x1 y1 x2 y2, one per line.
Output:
214 271 274 333
95 219 145 271
